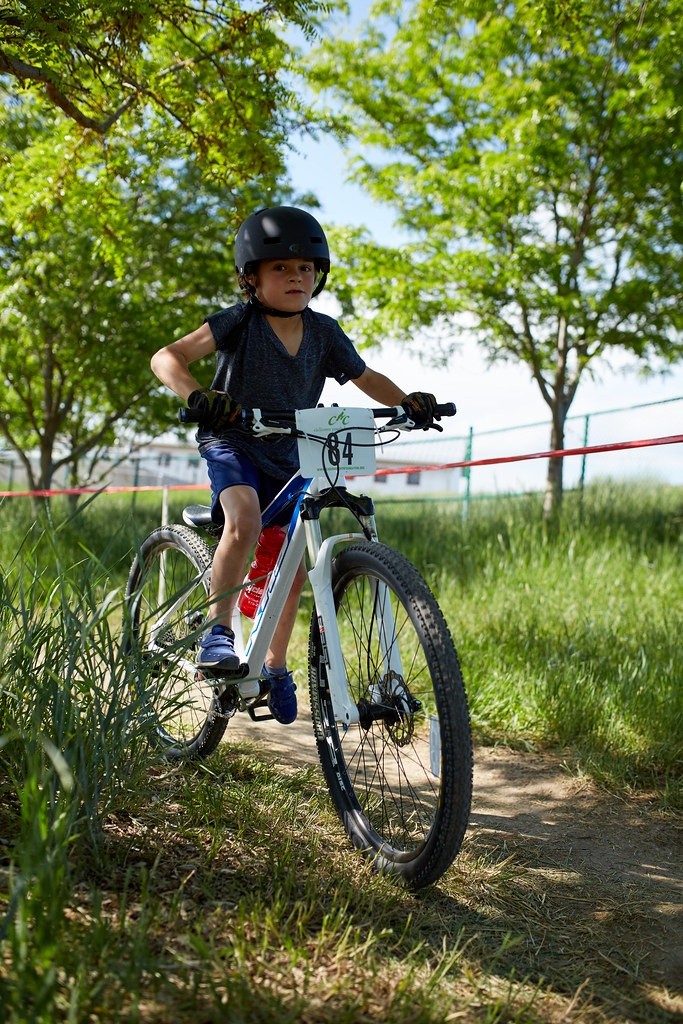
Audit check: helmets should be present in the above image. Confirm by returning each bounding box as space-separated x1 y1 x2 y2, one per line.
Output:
236 206 331 276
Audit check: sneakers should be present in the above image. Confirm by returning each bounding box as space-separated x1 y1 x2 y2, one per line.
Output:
194 623 240 669
261 662 298 725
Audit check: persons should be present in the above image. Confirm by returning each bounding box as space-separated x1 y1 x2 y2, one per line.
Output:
149 205 441 726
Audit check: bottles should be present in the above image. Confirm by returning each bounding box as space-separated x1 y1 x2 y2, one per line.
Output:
238 526 285 618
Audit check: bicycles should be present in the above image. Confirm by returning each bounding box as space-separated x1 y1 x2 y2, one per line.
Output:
119 400 474 891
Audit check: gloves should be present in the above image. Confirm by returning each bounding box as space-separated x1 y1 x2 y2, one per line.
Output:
187 387 238 427
401 392 441 424
